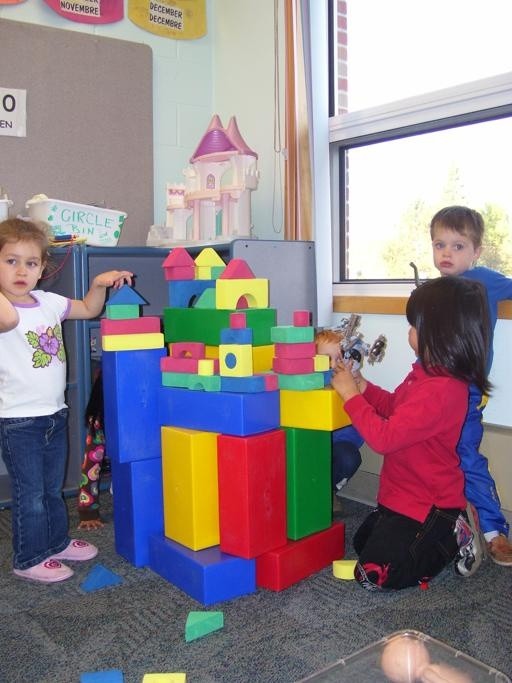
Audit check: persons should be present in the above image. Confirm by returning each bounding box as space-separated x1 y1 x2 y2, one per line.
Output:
314 328 365 494
0 216 135 581
76 371 106 532
429 205 510 568
328 276 496 589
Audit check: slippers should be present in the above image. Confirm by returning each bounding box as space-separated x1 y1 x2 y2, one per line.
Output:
12 555 75 583
53 537 99 561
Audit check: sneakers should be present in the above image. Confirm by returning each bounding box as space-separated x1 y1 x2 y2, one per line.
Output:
450 502 484 577
480 530 512 567
